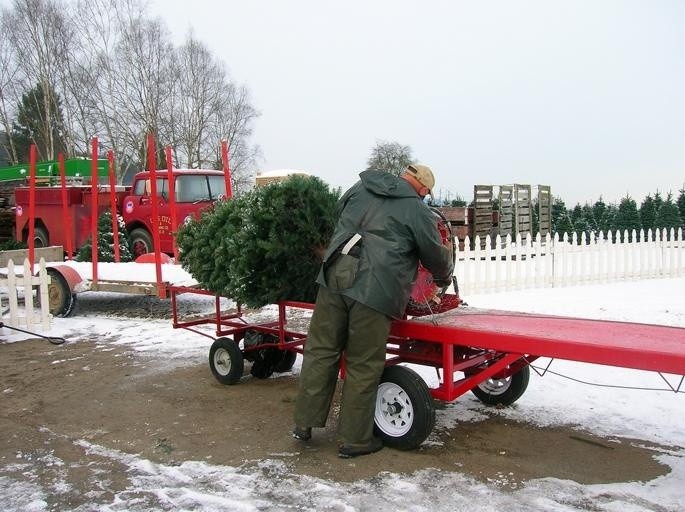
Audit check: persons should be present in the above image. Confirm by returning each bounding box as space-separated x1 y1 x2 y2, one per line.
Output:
291 161 453 458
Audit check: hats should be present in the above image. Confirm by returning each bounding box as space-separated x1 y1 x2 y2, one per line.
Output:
406 163 435 200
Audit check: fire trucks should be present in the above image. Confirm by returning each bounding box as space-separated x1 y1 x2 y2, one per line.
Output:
8 165 235 262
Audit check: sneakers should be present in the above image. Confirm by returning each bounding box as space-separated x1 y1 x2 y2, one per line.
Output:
340 438 384 458
292 426 312 441
